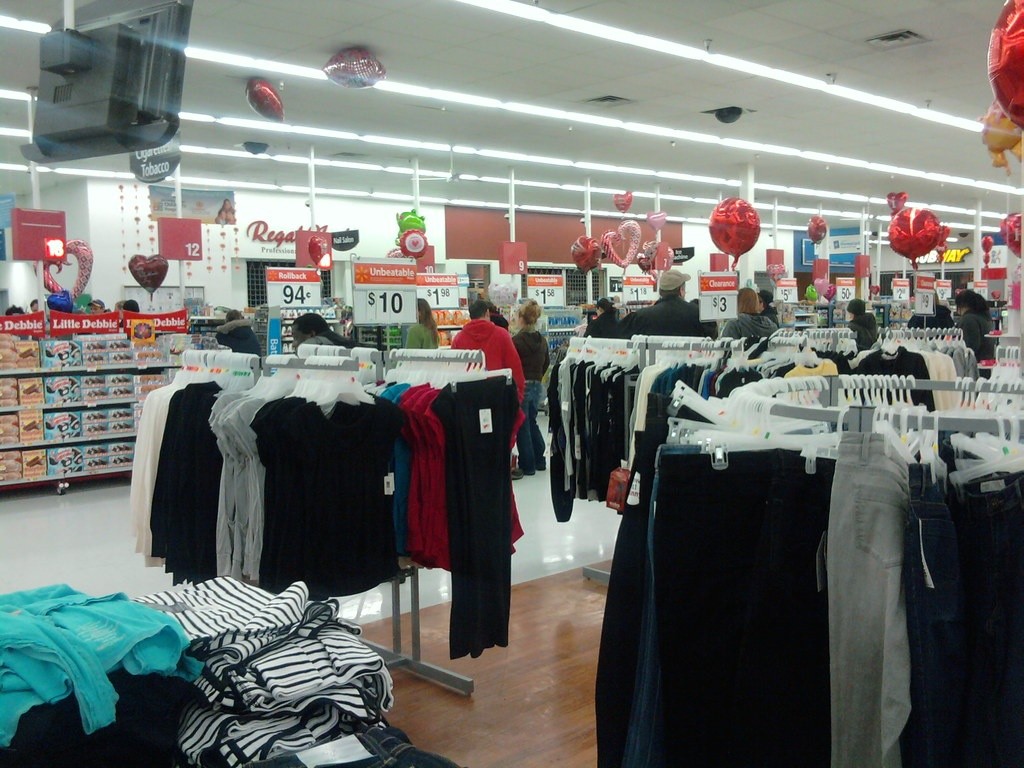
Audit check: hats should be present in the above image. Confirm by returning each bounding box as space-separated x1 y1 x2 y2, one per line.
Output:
659 270 691 290
87 299 105 309
758 290 773 304
847 298 865 315
123 300 139 312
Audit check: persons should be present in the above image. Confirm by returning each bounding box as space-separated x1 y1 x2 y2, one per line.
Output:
404 298 439 349
215 311 262 368
216 199 235 226
584 271 779 340
907 290 997 377
846 298 877 351
292 313 356 358
5 298 140 328
450 300 550 481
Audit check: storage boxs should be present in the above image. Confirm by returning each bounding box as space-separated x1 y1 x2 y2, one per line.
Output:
0 334 195 368
0 403 145 444
0 442 136 481
0 368 178 408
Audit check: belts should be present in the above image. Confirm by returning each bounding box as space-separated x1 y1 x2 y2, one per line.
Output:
524 379 540 384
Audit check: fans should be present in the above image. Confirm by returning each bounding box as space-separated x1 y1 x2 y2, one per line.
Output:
410 144 488 185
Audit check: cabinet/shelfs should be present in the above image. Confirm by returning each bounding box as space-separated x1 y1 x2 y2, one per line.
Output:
190 306 346 375
356 307 491 350
537 308 588 417
976 333 1021 369
0 361 184 496
777 301 911 329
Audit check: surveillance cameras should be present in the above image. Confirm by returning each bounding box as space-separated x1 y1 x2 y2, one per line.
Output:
580 218 585 223
305 199 309 207
714 107 742 124
243 141 269 154
504 214 509 219
960 233 969 237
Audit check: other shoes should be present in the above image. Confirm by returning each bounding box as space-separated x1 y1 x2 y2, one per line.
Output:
510 468 525 479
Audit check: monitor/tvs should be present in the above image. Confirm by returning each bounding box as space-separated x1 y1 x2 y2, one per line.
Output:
33 0 194 161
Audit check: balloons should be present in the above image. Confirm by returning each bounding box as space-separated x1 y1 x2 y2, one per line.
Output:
34 240 93 313
571 192 674 280
321 46 386 89
307 209 428 265
487 281 518 306
709 199 760 269
128 254 169 292
244 76 284 122
805 0 1024 302
766 263 786 285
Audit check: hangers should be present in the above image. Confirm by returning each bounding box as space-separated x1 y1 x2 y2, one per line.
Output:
172 342 513 417
559 327 970 373
663 345 1024 503
